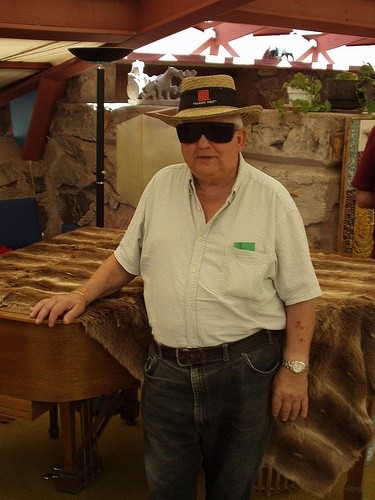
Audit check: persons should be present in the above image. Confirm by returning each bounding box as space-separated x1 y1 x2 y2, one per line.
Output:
30 75 324 500
351 125 375 259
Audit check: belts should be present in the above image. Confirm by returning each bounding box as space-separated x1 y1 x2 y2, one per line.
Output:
152 329 286 367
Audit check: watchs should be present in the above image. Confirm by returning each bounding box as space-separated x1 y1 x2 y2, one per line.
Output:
282 360 309 374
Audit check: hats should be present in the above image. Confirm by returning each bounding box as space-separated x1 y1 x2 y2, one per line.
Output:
156 75 263 128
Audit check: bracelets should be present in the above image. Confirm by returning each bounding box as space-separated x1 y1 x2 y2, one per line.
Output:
71 291 89 305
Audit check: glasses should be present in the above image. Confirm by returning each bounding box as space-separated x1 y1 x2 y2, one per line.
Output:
176 122 239 144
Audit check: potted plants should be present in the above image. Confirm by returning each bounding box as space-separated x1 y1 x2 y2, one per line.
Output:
325 63 375 115
270 72 332 114
